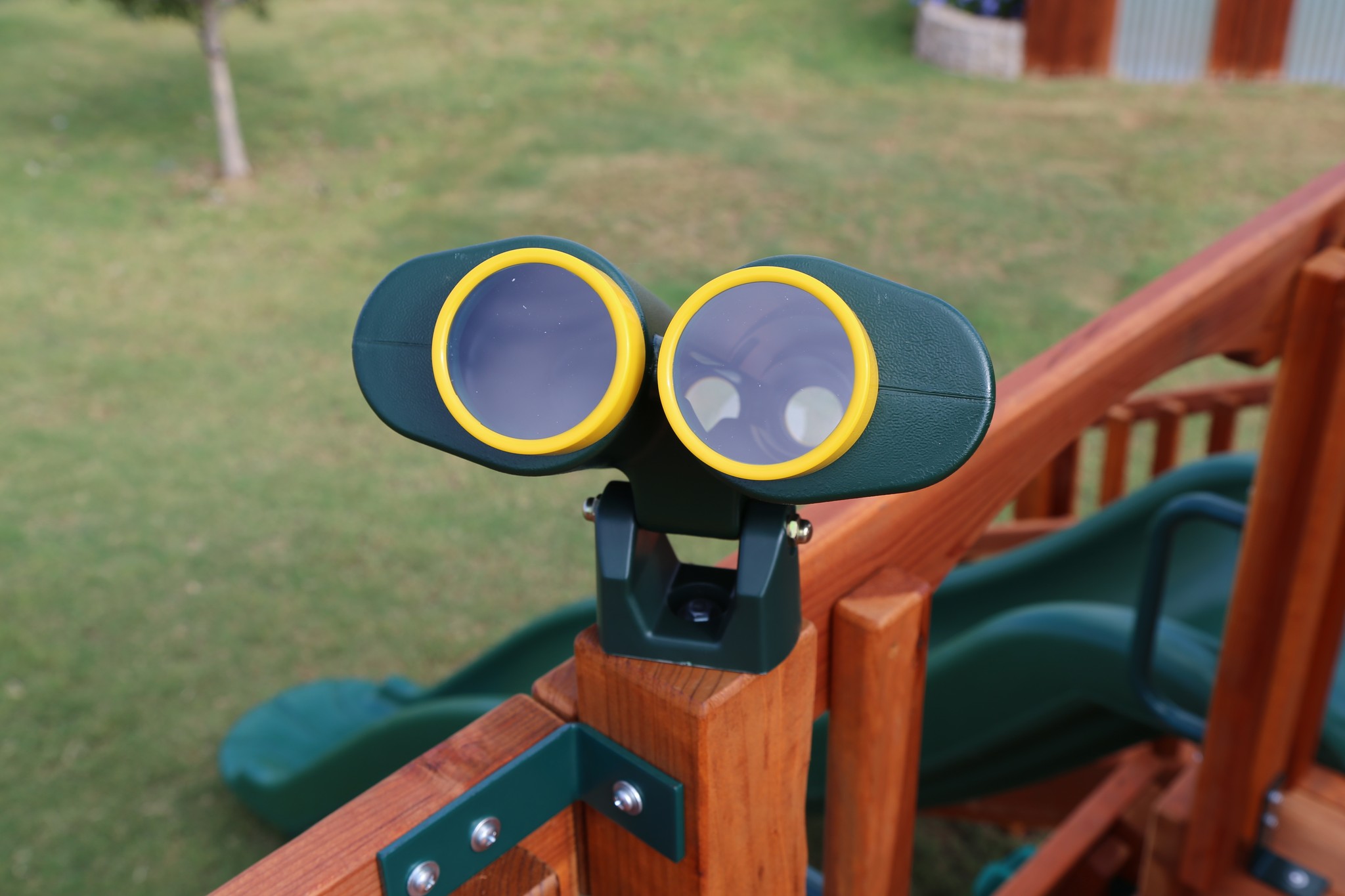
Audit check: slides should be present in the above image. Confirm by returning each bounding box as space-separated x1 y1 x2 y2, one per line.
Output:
215 449 1345 858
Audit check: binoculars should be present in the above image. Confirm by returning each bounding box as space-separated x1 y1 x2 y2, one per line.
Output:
350 233 996 507
